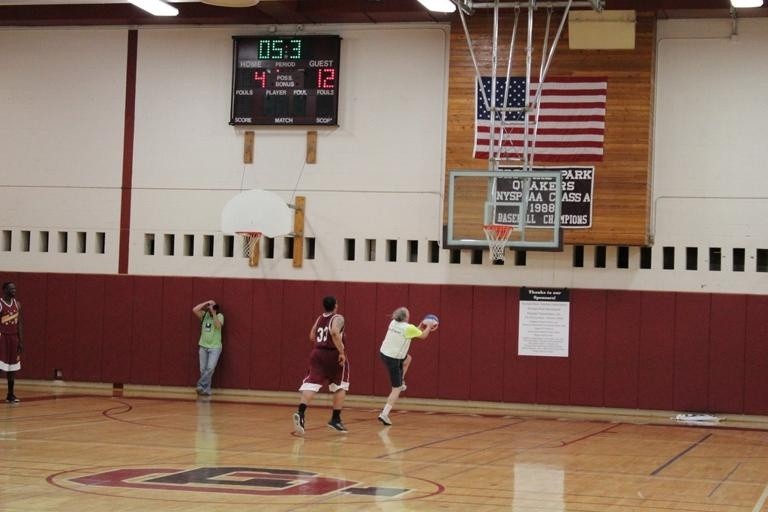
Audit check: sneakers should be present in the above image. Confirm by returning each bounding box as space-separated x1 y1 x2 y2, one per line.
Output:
401 379 407 391
5 393 20 402
378 412 392 425
196 388 210 395
327 420 348 433
292 413 305 435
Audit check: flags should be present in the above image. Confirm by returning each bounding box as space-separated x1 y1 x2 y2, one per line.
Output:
469 72 607 163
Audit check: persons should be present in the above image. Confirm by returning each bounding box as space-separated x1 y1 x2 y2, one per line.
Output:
378 306 439 426
0 280 25 405
293 292 352 435
191 299 226 396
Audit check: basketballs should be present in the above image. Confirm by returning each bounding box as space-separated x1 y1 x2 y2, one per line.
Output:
422 315 438 331
